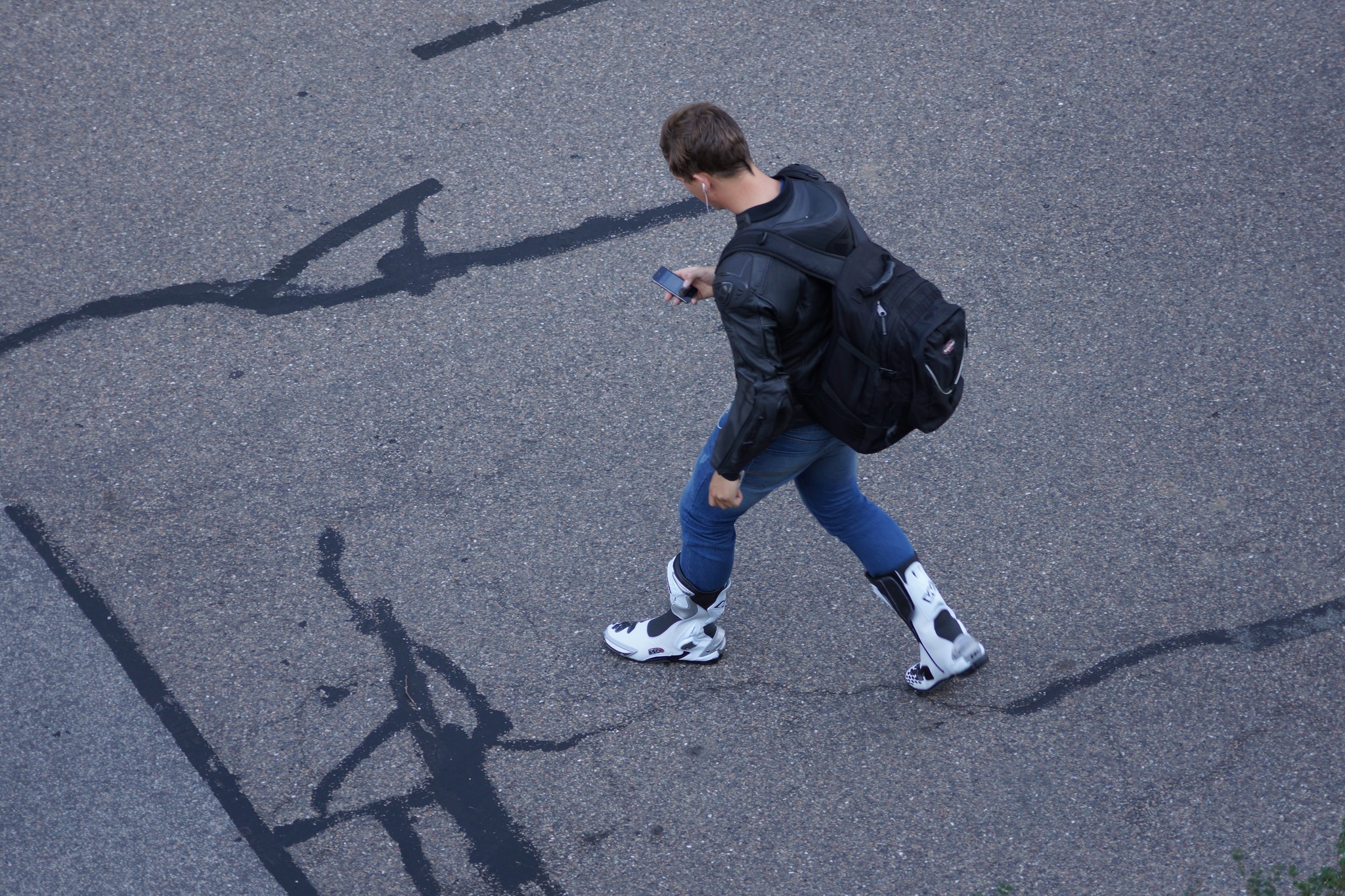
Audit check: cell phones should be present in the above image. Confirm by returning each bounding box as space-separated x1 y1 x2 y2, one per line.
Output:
651 266 698 304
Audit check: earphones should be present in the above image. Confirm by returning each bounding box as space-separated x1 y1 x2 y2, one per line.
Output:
702 183 705 192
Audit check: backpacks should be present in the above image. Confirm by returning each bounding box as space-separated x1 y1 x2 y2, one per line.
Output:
717 167 969 454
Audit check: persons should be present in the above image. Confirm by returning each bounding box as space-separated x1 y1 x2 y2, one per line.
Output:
596 100 993 696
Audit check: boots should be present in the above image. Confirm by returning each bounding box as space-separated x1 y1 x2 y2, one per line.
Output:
604 552 731 664
865 550 988 692
